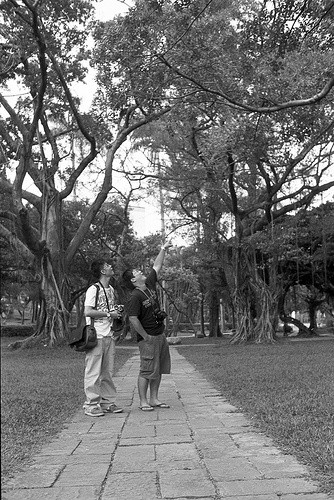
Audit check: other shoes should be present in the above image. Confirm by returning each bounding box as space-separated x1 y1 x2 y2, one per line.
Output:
83 401 124 416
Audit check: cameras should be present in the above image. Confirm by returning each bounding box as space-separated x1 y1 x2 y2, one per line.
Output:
151 308 168 324
114 304 124 312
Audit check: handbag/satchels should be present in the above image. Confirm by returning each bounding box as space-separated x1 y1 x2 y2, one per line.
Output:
69 325 99 352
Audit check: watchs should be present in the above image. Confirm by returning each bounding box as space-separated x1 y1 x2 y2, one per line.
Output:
107 313 110 317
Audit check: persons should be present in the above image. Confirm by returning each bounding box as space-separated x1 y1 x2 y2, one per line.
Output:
123 238 173 411
83 260 125 416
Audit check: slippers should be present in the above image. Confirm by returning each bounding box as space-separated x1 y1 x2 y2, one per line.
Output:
138 401 171 411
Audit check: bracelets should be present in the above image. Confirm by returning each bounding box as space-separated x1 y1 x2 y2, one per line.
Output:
161 248 167 251
